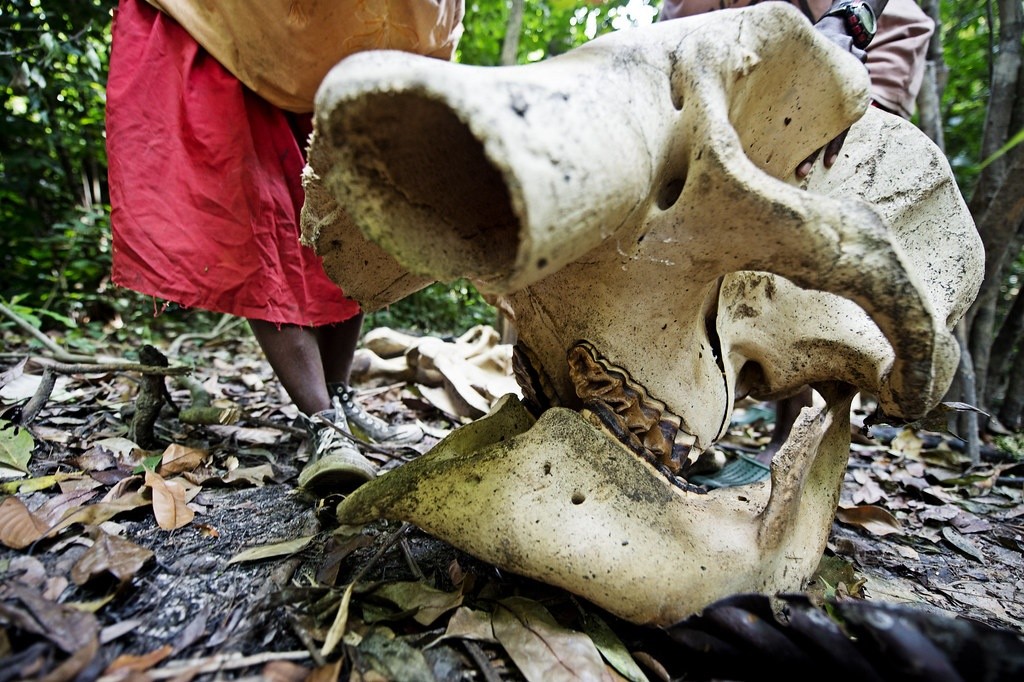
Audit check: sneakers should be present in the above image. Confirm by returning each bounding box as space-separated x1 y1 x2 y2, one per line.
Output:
294 382 425 495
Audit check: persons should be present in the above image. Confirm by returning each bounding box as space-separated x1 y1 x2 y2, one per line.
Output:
655 0 938 495
106 1 470 496
